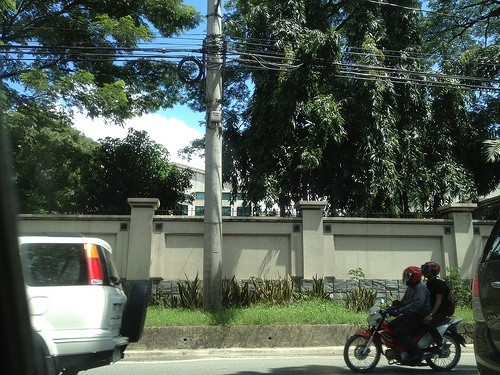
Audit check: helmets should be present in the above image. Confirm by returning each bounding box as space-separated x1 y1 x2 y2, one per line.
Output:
424 262 440 275
403 266 421 286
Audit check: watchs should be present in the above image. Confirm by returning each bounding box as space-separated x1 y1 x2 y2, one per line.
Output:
428 312 433 317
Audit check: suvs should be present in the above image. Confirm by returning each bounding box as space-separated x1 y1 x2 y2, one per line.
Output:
18 232 153 375
471 216 500 375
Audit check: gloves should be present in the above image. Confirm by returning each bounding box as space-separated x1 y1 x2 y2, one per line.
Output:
390 307 399 316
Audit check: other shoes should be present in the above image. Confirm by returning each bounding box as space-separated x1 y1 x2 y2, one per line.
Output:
407 352 421 361
438 338 445 350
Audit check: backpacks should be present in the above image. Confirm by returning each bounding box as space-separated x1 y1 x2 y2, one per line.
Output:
430 278 455 315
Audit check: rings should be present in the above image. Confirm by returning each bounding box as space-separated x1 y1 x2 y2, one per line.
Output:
426 319 427 320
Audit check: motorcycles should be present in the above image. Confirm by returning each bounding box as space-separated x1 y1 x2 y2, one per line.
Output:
344 299 467 372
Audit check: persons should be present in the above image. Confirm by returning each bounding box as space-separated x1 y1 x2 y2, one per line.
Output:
378 265 431 365
418 262 455 353
